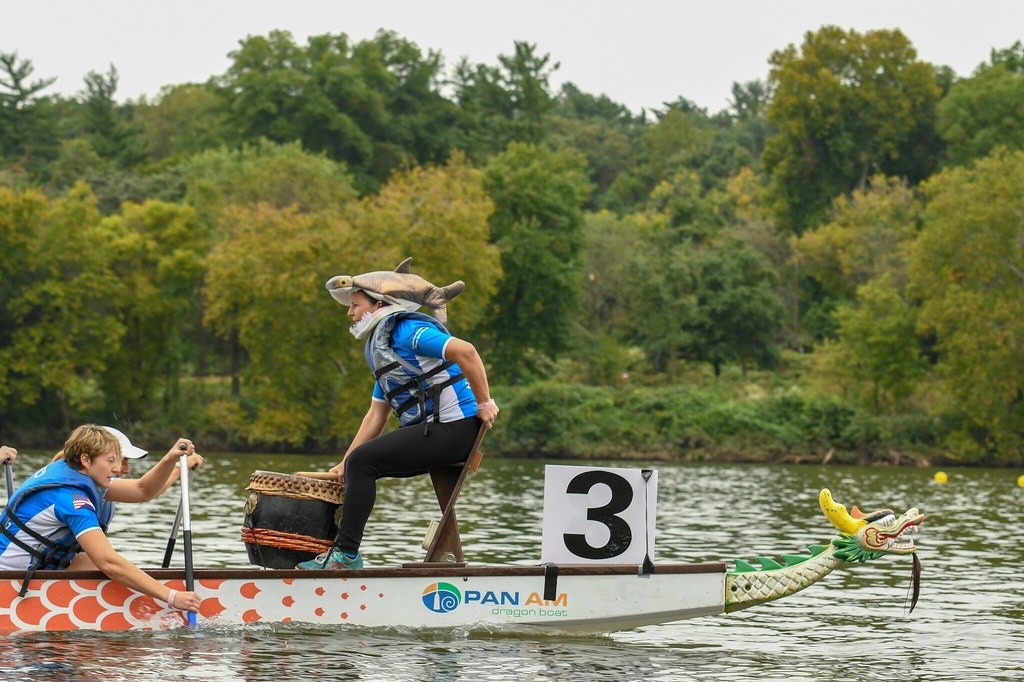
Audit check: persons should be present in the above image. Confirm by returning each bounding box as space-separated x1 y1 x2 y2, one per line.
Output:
295 256 500 571
0 425 203 614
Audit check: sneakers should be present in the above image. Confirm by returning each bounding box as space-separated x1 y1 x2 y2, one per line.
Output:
295 546 363 571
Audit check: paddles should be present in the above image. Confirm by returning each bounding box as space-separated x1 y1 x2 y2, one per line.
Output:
2 457 14 502
160 462 198 569
177 441 199 631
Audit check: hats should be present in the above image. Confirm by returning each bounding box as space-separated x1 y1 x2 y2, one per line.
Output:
99 426 148 459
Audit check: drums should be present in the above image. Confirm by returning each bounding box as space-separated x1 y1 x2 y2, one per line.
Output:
240 468 348 571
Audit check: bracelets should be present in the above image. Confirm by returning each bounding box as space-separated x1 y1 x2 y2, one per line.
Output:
176 461 182 468
476 399 495 410
169 588 177 610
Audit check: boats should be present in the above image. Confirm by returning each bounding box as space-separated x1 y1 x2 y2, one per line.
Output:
0 461 930 637
